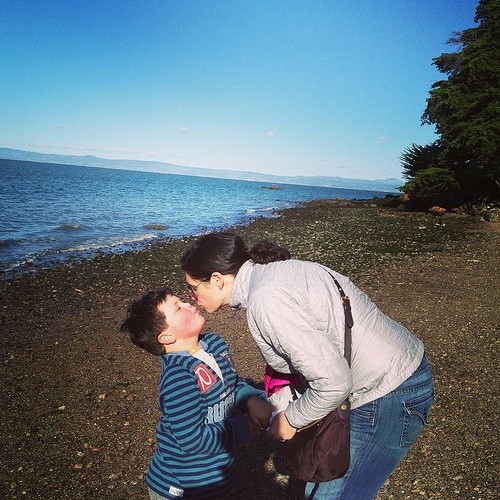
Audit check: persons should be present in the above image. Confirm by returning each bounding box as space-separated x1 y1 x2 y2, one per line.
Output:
116 283 282 500
172 232 434 500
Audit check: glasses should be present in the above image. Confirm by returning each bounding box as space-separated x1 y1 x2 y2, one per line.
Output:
187 274 209 295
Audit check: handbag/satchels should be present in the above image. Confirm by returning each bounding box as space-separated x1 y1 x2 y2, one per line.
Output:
268 386 351 483
